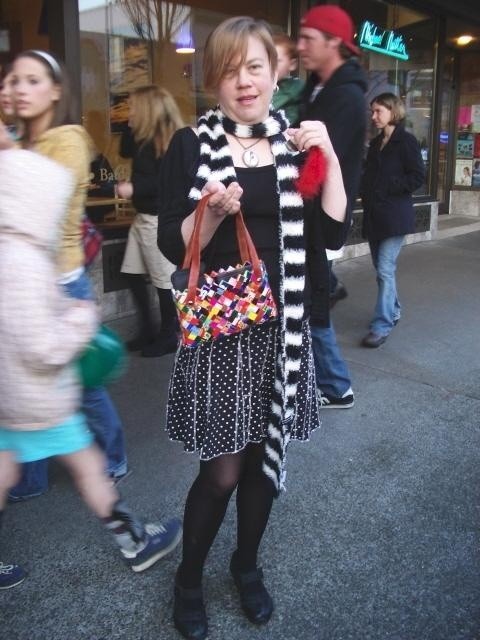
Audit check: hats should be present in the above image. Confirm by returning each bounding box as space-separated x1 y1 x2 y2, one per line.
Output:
300 6 360 53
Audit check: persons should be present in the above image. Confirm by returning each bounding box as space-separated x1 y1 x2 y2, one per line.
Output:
296 3 366 409
0 148 184 593
2 48 129 498
266 38 304 125
156 16 348 640
328 244 348 311
458 166 472 186
116 84 185 357
359 93 426 348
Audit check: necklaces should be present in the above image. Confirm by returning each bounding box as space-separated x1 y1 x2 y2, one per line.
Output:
227 135 271 166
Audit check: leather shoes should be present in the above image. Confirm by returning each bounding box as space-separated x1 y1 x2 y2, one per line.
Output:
329 282 348 308
174 583 207 639
362 331 386 348
230 551 273 625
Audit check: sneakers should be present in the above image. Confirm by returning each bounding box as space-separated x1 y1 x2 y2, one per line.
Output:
142 335 178 357
121 518 184 573
0 561 26 590
317 386 355 409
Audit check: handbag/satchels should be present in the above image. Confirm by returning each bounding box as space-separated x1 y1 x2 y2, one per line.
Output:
170 197 278 345
84 326 122 388
